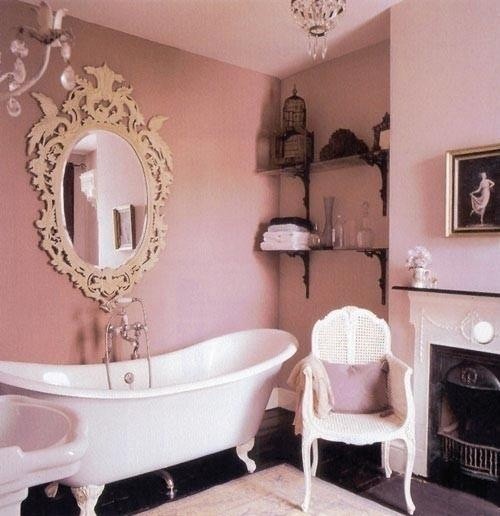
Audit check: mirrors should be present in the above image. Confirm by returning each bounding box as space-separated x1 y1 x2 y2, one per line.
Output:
25 61 172 313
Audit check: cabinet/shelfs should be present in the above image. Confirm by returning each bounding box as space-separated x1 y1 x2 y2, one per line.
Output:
257 147 390 306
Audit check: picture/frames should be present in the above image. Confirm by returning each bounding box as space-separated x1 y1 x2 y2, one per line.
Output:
112 204 135 252
445 143 500 238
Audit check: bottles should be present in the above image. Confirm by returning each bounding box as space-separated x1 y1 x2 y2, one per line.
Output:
312 196 376 251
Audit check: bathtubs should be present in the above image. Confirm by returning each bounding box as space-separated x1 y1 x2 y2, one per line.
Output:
0 328 302 486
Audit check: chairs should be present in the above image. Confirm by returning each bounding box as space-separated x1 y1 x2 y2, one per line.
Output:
300 305 417 515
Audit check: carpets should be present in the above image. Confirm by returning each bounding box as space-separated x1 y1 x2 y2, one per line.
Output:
130 463 408 516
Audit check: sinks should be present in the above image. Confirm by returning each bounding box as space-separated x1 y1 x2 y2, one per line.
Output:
0 394 87 494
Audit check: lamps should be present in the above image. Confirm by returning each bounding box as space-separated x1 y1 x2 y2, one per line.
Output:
291 1 346 59
0 0 76 116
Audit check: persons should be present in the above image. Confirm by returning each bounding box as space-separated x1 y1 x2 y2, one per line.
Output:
469 171 496 224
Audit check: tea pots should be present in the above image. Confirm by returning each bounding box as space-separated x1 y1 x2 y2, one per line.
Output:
410 267 430 289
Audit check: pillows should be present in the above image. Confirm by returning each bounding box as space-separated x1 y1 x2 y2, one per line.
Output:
320 359 390 414
